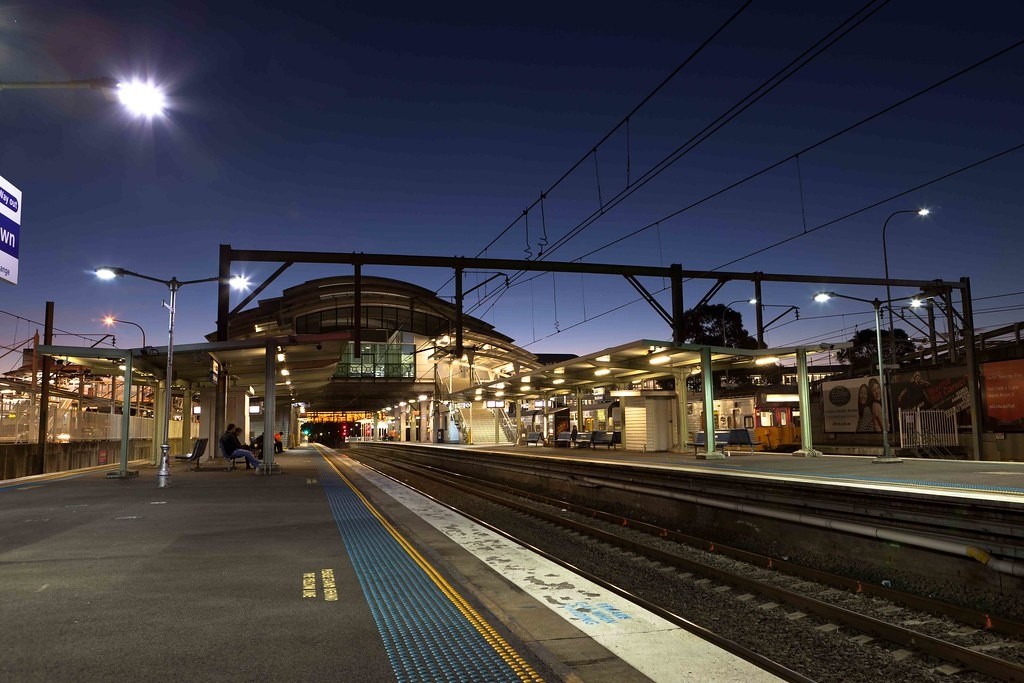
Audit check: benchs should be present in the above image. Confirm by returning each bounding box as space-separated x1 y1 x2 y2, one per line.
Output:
219 437 276 471
592 431 617 451
551 432 572 448
715 428 762 456
523 432 540 447
687 431 731 457
574 432 592 449
175 437 208 471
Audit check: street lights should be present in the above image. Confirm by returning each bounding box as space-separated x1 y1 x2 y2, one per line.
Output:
810 292 935 455
882 206 931 362
722 298 756 350
94 266 252 488
104 317 147 347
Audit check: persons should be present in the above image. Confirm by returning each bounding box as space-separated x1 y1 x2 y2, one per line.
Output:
234 428 255 468
898 371 930 408
868 378 883 431
855 383 873 432
253 431 285 460
221 423 263 467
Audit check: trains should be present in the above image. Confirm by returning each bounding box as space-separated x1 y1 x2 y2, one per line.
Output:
379 382 805 456
